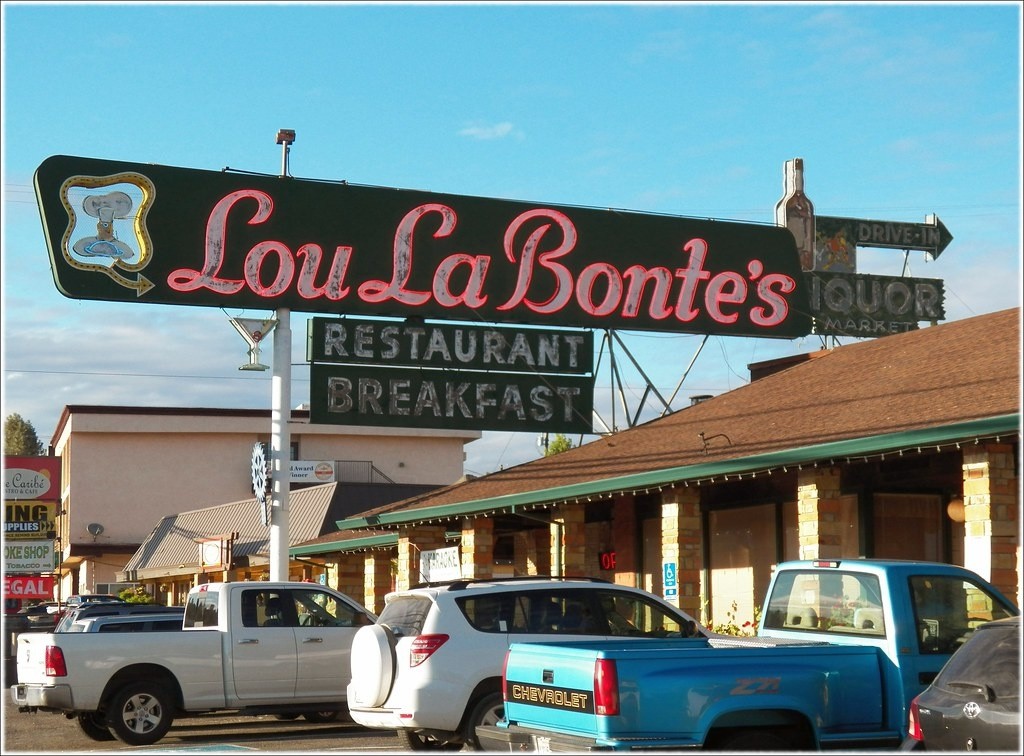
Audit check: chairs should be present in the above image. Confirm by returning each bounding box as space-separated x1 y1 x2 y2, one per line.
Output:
828 608 884 635
544 602 562 634
562 605 582 634
262 598 283 626
783 607 821 630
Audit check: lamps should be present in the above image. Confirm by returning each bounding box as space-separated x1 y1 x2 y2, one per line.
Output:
946 494 965 523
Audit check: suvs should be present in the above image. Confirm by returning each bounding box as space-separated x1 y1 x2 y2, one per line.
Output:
345 573 742 752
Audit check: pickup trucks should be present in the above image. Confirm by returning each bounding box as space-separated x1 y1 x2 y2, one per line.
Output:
492 556 1020 752
9 579 389 746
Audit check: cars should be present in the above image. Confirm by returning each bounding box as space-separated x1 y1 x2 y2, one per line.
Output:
6 592 187 648
905 615 1020 751
